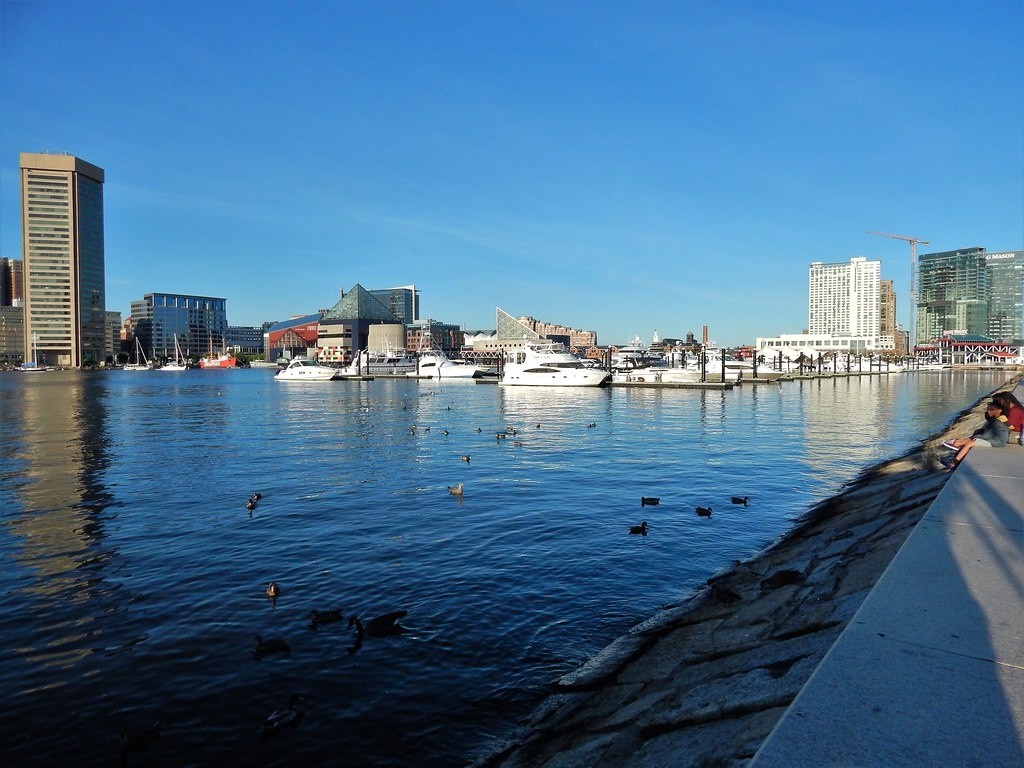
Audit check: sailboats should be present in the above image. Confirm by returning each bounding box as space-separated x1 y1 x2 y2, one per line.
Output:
124 334 237 372
405 333 490 378
342 321 421 375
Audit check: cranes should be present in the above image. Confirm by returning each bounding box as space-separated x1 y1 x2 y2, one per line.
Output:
867 230 930 358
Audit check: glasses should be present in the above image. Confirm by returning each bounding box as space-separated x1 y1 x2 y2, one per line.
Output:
987 407 998 411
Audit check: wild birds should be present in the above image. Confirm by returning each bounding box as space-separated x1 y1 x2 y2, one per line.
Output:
586 421 597 429
445 479 467 497
493 422 541 448
460 454 472 463
115 718 167 758
255 691 305 735
731 496 751 505
626 520 649 535
410 423 450 437
252 632 293 655
695 505 713 517
306 607 415 639
641 496 661 504
245 492 262 511
476 427 482 433
402 389 455 412
266 580 281 600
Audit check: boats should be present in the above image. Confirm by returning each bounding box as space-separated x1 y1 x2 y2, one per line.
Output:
687 354 738 370
647 342 665 358
274 359 339 380
611 354 700 383
611 335 649 360
700 360 786 382
497 343 611 387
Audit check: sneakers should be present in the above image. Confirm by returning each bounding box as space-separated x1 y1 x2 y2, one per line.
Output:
940 457 956 469
942 438 961 450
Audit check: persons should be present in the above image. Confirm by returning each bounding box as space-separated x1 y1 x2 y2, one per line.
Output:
943 391 1024 451
940 401 1008 469
